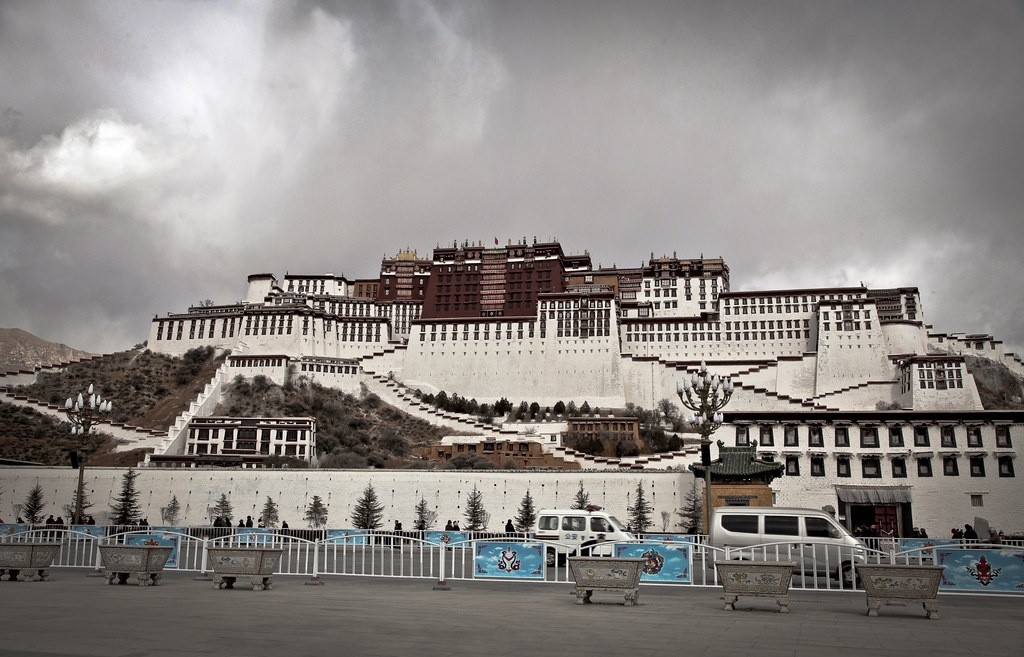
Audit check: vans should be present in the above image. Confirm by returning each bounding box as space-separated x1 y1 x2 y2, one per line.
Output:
707 506 869 588
534 503 637 567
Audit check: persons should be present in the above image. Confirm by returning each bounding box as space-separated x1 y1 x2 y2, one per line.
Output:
504 519 517 537
853 525 1002 561
17 514 291 546
393 519 463 551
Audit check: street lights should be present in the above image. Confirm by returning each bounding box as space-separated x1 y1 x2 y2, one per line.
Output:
65 383 112 525
677 359 734 534
